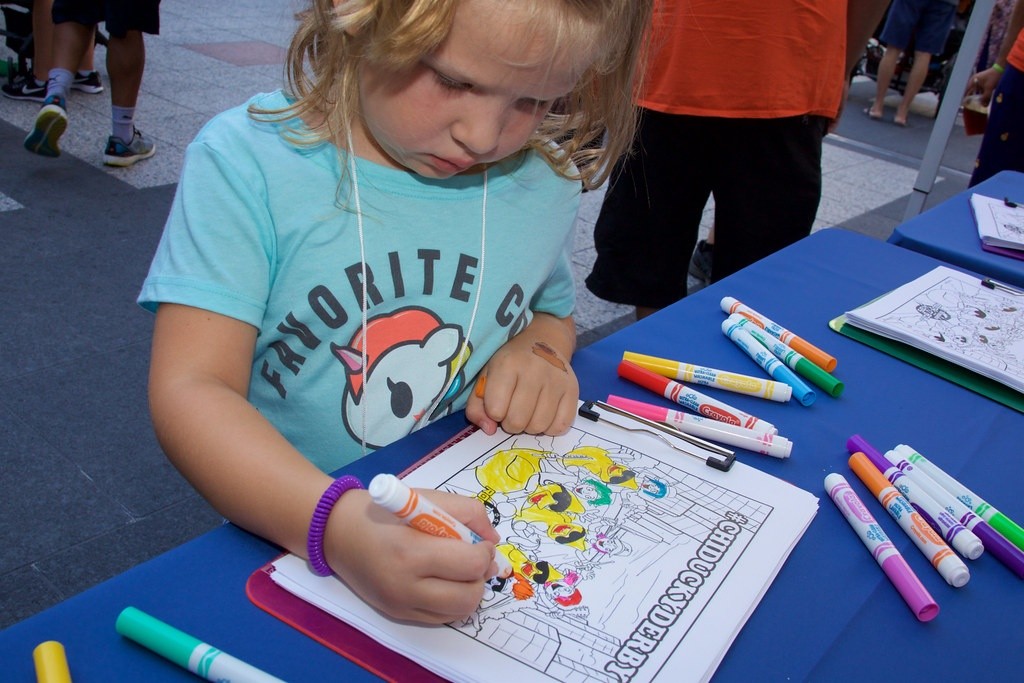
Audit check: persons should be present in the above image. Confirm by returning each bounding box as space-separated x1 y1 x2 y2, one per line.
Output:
2 0 103 102
23 0 162 168
869 0 1024 190
135 0 650 626
585 0 888 319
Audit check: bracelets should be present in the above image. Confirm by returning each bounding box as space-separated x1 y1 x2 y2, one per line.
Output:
992 62 1005 74
305 475 366 576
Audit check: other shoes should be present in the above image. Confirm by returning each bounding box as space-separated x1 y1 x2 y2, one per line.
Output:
691 240 715 282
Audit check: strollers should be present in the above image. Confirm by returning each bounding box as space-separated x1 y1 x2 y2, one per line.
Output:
850 0 967 119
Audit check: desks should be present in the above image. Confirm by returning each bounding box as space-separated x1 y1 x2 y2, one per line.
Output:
889 171 1024 288
1 227 1024 682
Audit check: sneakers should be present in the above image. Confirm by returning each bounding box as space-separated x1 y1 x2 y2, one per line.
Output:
1 73 48 102
102 125 155 167
24 94 68 158
71 70 104 94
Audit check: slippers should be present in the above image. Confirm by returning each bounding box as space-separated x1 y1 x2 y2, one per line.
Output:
892 114 911 127
863 106 883 119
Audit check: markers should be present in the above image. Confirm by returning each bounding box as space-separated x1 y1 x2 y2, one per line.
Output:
32 640 72 683
721 296 839 374
823 472 941 623
367 471 522 581
882 450 1024 581
116 606 289 683
723 319 819 407
847 433 984 560
618 360 780 436
849 452 972 587
606 395 793 460
622 349 801 403
728 311 846 398
893 444 1024 551
